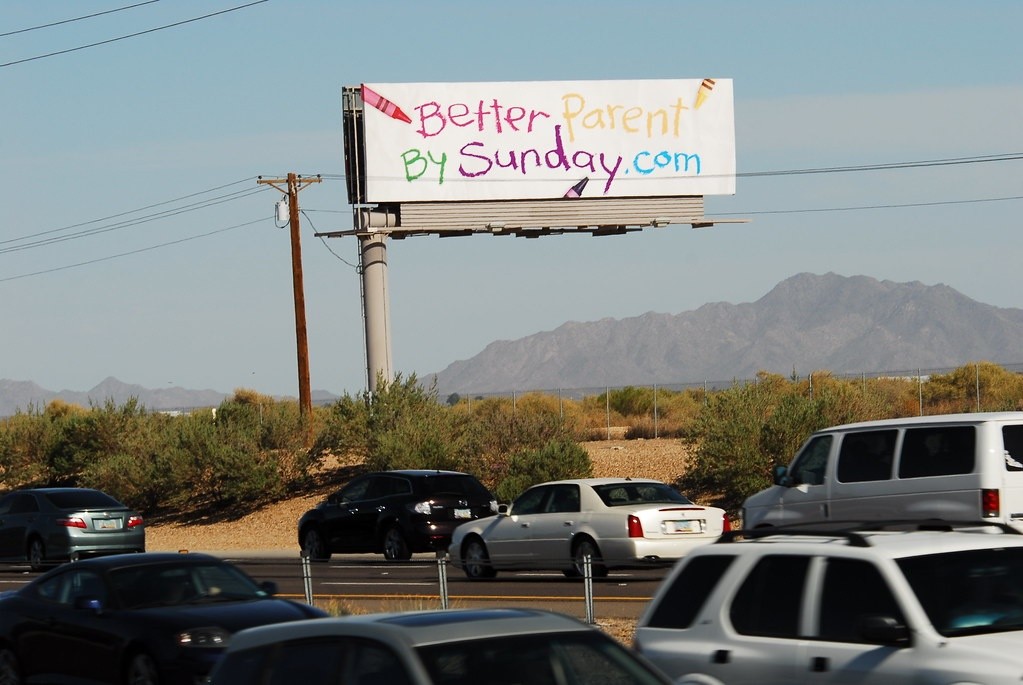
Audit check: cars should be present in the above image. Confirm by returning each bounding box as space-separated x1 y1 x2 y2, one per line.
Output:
447 478 732 582
0 486 146 573
205 606 673 685
0 553 332 685
298 470 503 563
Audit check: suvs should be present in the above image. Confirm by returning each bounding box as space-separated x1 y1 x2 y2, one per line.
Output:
631 516 1023 685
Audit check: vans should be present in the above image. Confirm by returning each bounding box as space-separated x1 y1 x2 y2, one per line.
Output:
741 410 1023 537
